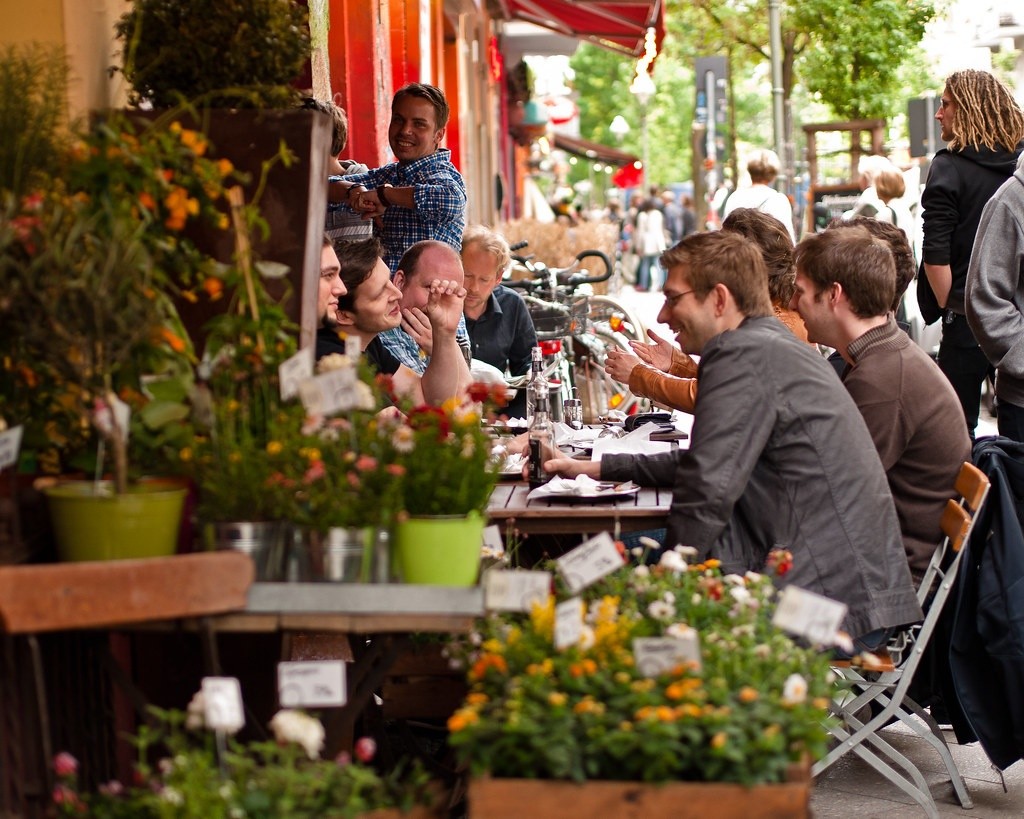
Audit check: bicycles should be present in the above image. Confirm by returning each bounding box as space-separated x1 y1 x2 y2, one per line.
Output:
500 241 647 425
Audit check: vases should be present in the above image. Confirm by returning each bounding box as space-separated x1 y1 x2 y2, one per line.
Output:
288 521 389 584
468 758 812 819
43 478 188 561
206 521 283 582
396 515 483 587
91 107 333 379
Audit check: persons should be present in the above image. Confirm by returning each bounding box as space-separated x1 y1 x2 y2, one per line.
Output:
315 239 473 408
327 82 473 378
917 70 1024 442
323 103 373 240
522 149 972 661
964 151 1024 444
459 223 537 438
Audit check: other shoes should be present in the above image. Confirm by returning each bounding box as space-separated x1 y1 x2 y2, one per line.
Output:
635 285 648 292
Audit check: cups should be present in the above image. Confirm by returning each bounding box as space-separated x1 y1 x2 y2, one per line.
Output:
577 375 607 421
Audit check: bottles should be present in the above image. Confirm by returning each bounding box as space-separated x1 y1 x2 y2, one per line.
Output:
486 444 508 472
528 384 555 491
527 347 549 428
564 399 583 431
599 426 626 440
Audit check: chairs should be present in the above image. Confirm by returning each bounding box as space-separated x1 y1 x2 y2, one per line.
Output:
811 462 992 819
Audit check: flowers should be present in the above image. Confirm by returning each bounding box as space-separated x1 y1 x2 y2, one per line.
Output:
0 0 835 819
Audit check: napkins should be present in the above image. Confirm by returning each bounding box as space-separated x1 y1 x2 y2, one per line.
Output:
492 415 529 427
490 445 529 471
526 472 634 499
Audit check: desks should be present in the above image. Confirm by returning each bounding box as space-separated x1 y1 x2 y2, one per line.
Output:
483 437 678 534
0 550 252 790
138 579 484 764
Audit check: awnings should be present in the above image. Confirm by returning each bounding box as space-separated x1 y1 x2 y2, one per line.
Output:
550 133 641 188
500 0 666 76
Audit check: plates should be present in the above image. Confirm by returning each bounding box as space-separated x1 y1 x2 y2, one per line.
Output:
561 481 640 500
488 471 522 479
600 418 625 424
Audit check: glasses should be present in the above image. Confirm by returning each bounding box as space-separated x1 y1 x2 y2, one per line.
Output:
665 289 695 308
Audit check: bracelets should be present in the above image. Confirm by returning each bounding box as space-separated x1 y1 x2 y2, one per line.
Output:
377 184 393 208
344 183 365 202
